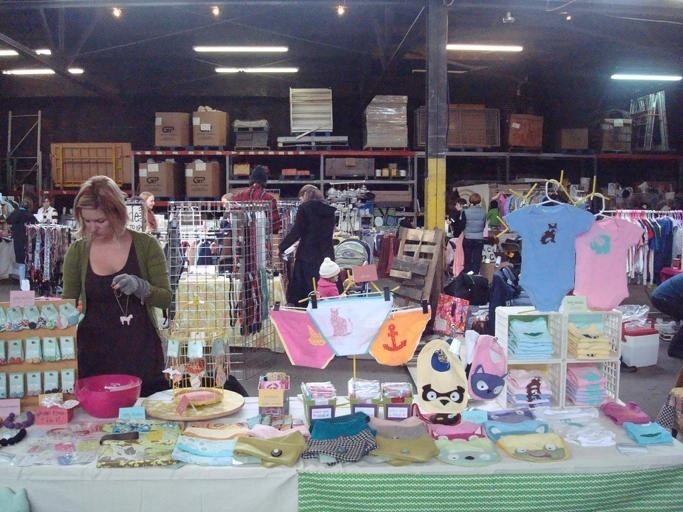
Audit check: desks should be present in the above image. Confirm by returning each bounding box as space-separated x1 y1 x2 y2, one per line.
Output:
1 396 682 511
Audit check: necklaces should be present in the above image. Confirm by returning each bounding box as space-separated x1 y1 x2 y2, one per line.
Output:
113 286 134 326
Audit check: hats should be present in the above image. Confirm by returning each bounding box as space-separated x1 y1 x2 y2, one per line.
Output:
319 257 341 279
249 164 269 183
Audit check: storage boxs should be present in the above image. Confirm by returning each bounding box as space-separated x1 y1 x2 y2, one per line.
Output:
549 126 588 154
184 161 221 200
153 112 188 147
191 112 232 148
324 158 375 177
621 318 660 367
446 104 487 151
138 161 179 199
502 114 544 152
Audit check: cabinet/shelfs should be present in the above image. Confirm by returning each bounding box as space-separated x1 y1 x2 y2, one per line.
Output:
227 153 416 245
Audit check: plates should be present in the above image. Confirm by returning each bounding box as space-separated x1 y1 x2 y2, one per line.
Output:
144 389 245 422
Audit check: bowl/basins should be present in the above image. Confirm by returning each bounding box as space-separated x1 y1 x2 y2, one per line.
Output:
74 375 142 419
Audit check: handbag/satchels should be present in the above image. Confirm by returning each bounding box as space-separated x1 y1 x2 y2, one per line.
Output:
432 265 524 337
459 212 466 231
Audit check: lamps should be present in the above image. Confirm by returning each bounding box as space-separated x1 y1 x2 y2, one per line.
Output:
2 67 82 77
215 66 299 73
0 48 51 56
447 42 526 53
611 73 683 83
192 44 289 54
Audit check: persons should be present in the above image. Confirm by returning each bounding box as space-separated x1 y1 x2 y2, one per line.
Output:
139 190 158 229
220 192 236 229
315 255 347 301
60 176 172 397
230 164 281 264
35 196 58 226
485 200 502 231
5 201 38 265
275 184 336 309
650 271 682 359
461 192 487 276
449 198 468 237
592 186 683 211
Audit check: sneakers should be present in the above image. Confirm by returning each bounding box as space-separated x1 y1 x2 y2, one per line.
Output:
621 360 637 372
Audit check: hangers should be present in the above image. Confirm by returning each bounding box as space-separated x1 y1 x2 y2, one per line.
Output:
534 179 683 221
271 272 428 314
